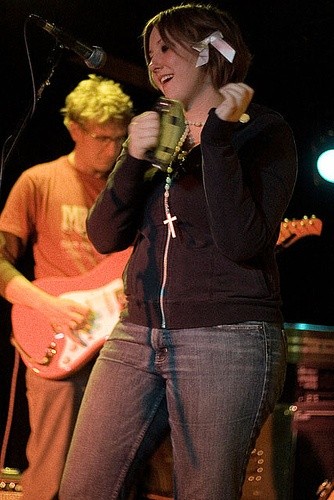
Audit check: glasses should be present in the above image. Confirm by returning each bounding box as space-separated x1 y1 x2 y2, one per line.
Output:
69 117 128 144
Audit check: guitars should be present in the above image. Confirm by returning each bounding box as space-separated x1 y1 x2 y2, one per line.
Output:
11 213 321 380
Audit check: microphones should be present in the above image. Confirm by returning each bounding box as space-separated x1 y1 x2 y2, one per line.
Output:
28 13 106 70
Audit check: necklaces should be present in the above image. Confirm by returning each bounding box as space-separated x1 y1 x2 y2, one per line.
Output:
162 119 207 238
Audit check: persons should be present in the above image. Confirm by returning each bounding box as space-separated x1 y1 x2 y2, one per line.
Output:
58 3 298 500
2 74 135 500
